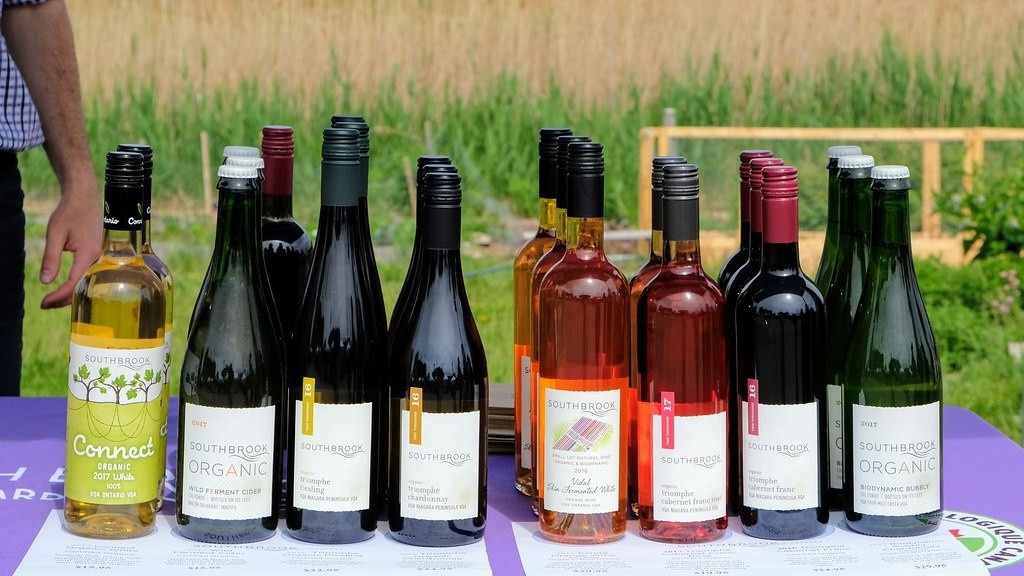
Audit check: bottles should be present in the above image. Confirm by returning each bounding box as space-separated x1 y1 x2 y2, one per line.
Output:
66 116 944 546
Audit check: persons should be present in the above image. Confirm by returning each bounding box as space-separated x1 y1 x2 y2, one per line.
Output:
0 0 103 397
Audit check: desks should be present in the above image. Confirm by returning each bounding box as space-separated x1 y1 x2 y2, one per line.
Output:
0 395 1024 576
